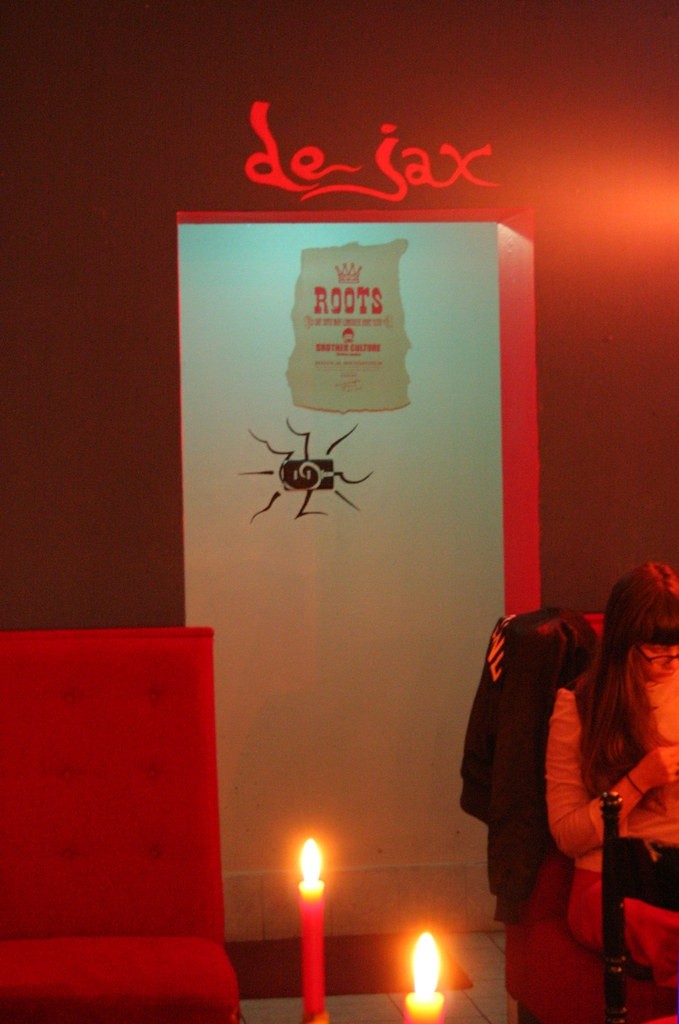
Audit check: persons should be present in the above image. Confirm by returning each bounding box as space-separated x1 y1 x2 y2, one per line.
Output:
545 561 678 986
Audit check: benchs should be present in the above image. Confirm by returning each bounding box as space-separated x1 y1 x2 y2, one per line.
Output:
0 627 240 1023
504 614 679 1023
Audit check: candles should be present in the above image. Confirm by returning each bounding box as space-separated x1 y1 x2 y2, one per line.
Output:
401 930 448 1023
295 837 325 1024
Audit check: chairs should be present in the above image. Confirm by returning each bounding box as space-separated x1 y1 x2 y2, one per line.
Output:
600 790 679 1023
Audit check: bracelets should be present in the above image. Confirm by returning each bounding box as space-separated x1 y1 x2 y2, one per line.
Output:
626 772 646 796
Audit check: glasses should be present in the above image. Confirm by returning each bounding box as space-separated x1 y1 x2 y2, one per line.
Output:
634 643 679 667
